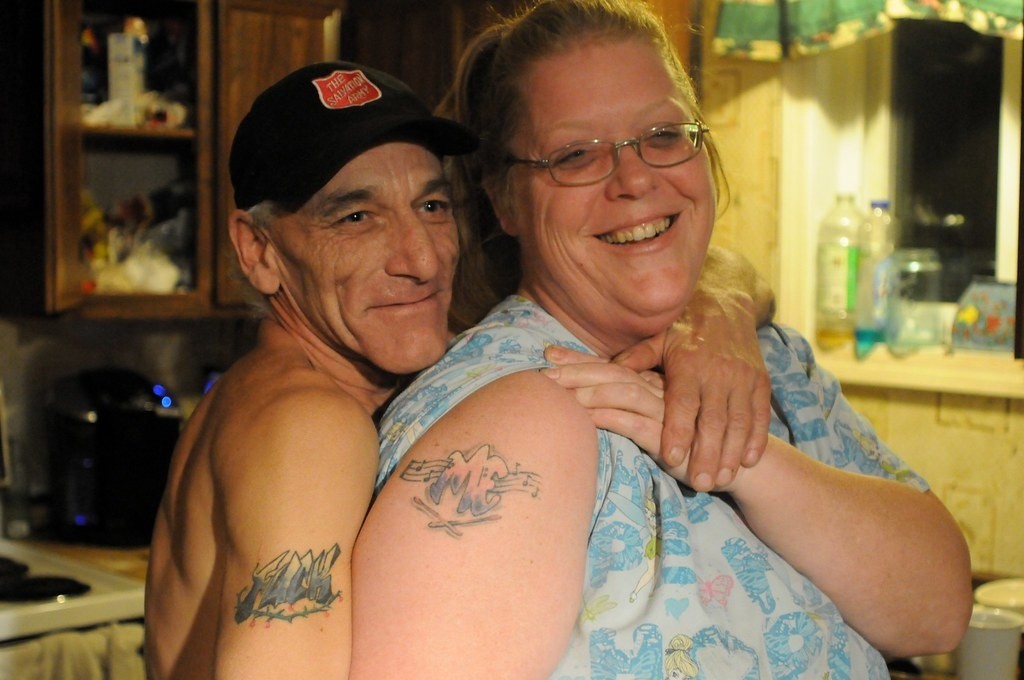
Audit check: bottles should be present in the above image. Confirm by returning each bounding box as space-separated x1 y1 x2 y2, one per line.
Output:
814 190 856 352
854 201 900 359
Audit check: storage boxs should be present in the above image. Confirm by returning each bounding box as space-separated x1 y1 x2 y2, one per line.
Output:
108 33 147 103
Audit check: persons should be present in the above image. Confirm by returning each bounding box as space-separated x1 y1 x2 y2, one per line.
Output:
146 61 775 679
349 1 974 680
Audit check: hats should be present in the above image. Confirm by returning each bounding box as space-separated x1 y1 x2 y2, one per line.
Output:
229 62 480 213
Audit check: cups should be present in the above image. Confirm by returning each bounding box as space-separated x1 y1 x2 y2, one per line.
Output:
973 578 1023 615
887 249 942 356
956 605 1023 680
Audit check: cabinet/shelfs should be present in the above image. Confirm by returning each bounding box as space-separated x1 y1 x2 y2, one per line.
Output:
0 0 693 317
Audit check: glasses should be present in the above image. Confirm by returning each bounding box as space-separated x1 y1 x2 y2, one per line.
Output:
505 119 709 186
